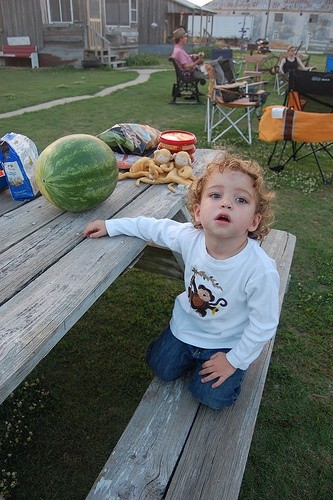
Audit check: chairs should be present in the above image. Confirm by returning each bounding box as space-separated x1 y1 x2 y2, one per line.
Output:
168 41 333 182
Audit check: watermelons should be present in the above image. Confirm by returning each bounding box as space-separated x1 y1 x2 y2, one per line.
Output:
35 133 116 213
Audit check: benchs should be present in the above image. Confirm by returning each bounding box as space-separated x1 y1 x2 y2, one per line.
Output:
85 229 297 500
0 44 39 68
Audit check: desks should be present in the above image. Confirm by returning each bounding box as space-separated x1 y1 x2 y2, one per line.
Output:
1 148 226 403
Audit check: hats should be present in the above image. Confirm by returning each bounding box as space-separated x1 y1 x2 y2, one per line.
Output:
172 28 188 40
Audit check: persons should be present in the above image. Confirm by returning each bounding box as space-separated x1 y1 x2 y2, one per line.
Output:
279 45 311 75
84 154 281 411
172 27 209 79
